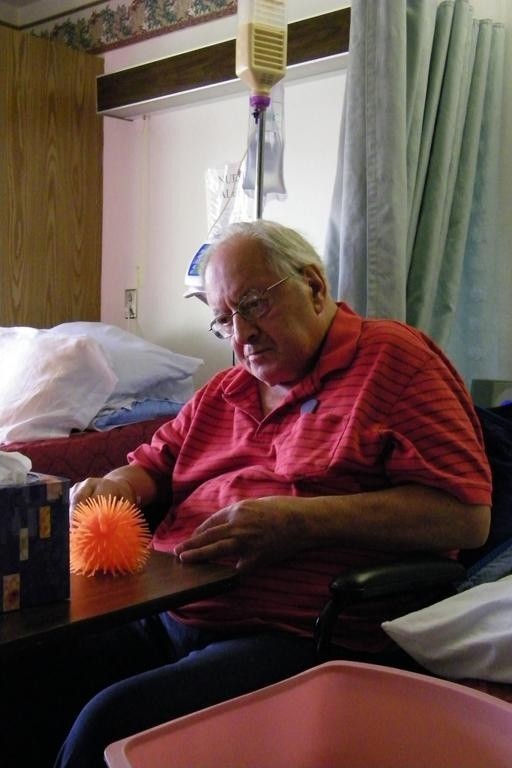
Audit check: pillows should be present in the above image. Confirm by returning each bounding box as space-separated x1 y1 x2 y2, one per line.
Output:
40 320 205 400
0 326 117 446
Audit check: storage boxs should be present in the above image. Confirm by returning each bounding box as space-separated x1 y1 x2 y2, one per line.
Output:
102 659 511 768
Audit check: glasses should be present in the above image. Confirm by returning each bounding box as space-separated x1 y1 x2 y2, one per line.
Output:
208 276 290 340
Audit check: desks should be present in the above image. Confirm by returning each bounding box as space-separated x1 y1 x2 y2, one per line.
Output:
0 548 244 768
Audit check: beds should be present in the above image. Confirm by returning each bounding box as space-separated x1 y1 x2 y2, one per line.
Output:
0 417 174 491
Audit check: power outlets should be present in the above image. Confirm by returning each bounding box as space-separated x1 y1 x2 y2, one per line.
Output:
123 289 136 319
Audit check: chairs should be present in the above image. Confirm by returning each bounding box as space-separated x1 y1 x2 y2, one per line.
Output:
313 400 511 670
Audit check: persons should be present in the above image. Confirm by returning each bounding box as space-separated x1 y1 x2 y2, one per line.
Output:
1 220 495 768
